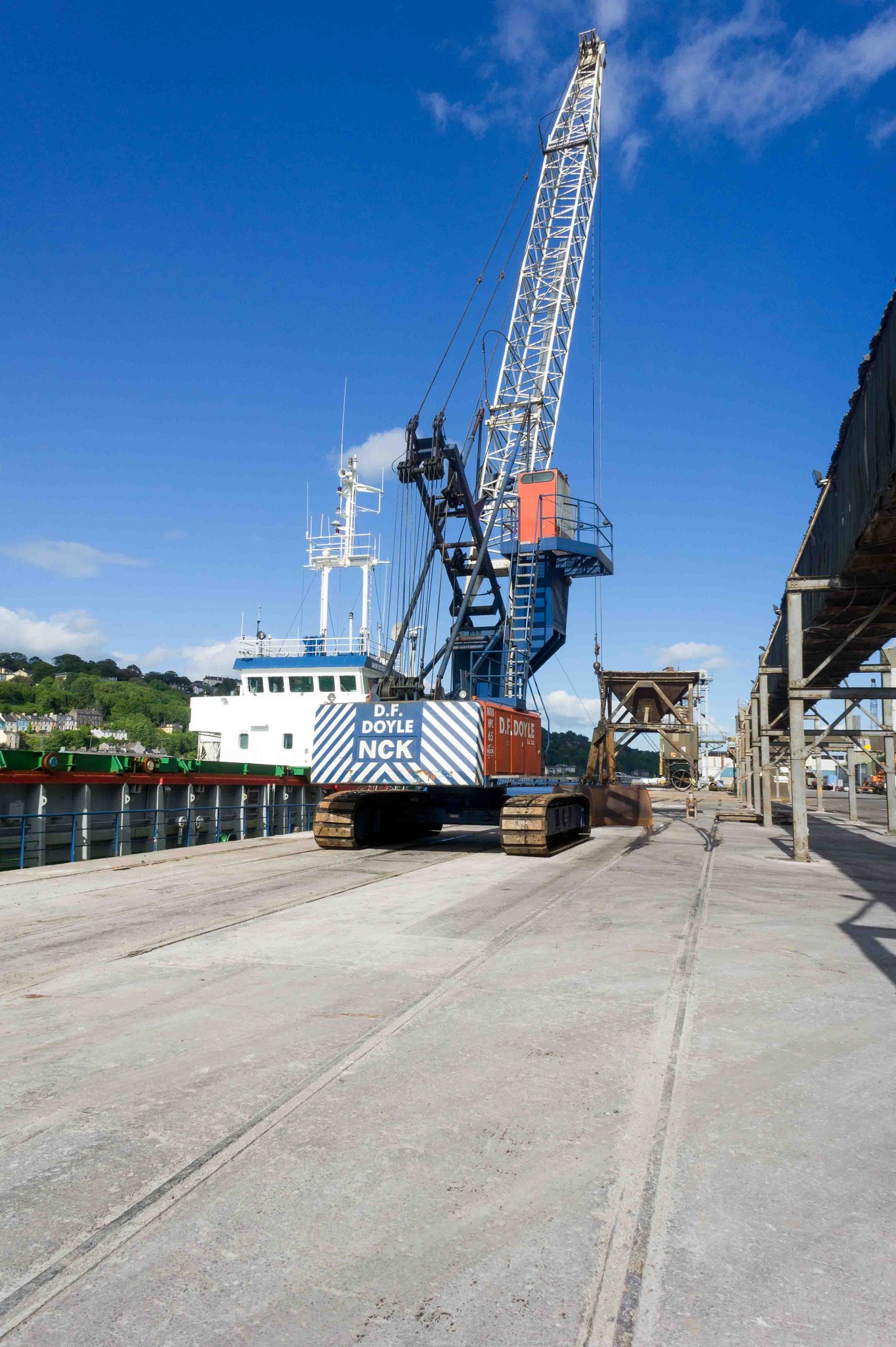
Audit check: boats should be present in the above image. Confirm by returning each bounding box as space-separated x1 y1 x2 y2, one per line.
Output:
0 453 429 871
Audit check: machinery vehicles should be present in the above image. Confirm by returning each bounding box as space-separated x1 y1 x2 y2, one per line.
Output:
856 762 885 794
311 27 619 859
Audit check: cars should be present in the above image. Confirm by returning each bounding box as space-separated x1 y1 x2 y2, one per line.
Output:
835 786 842 791
843 786 849 791
